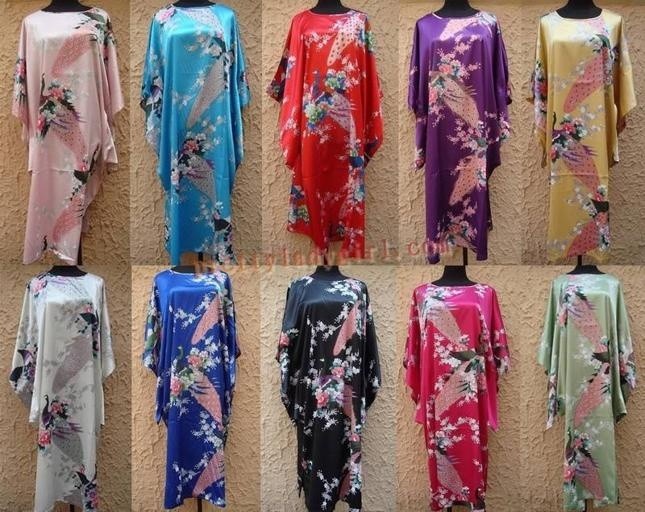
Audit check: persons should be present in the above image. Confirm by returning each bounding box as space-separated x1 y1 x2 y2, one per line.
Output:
140 1 249 267
11 1 124 267
403 264 510 511
531 2 638 264
266 1 384 261
275 265 381 511
537 263 640 511
141 266 240 511
10 268 115 511
406 1 512 265
